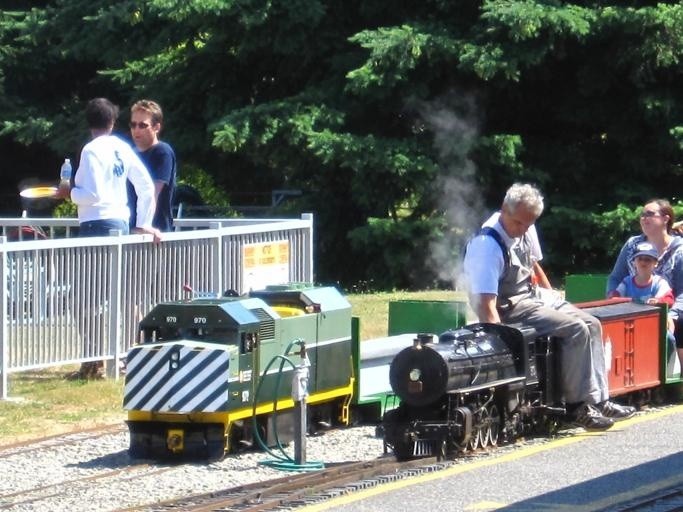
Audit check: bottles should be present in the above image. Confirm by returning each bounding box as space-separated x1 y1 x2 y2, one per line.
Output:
60 158 72 186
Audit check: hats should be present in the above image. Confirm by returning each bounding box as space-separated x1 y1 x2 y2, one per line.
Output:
626 240 660 263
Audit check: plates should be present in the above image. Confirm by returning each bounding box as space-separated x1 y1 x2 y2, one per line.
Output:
18 187 58 199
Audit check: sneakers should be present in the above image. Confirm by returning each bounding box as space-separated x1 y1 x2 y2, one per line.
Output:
566 402 615 432
600 400 637 421
62 367 107 382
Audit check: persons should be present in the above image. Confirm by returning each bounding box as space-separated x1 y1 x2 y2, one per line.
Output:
461 182 638 432
610 242 675 369
604 197 682 382
51 95 161 383
117 99 180 376
